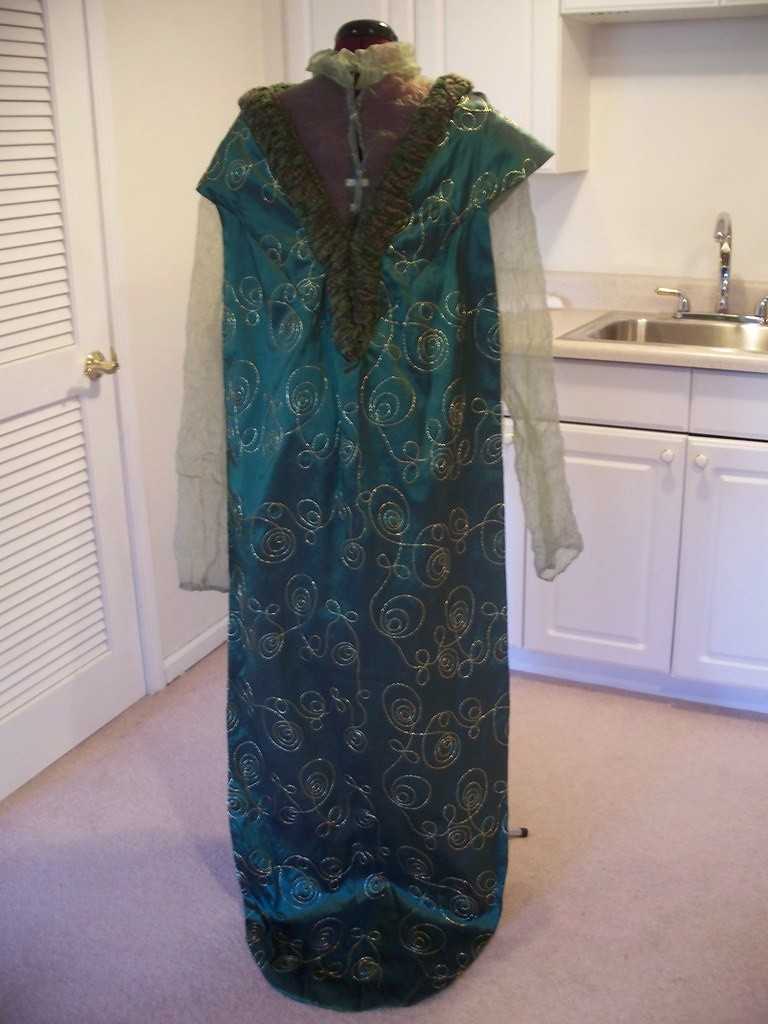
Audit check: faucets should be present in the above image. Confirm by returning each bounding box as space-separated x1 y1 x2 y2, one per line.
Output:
713 213 732 313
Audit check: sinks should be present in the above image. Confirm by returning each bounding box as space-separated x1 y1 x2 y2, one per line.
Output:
557 311 768 354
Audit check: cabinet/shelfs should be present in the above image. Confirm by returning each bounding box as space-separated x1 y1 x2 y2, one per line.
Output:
496 268 768 719
285 0 590 177
